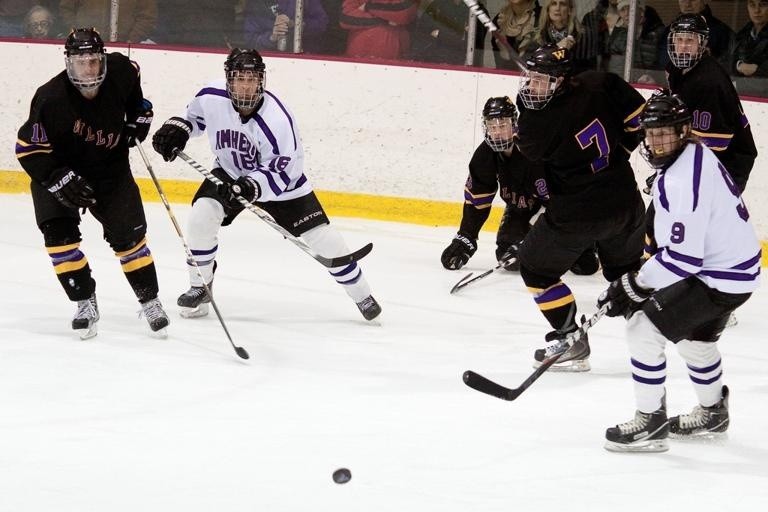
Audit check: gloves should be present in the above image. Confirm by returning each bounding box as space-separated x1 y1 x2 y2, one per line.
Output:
441 232 477 270
128 110 154 147
217 176 261 209
598 271 651 317
51 171 96 209
152 117 193 162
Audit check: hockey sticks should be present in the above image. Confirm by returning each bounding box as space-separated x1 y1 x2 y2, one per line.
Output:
452 255 522 295
171 147 373 268
133 136 249 361
463 300 616 402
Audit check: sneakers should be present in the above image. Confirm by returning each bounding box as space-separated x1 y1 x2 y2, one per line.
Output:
356 295 381 321
142 297 168 331
606 408 668 443
667 400 729 434
177 283 212 307
535 332 590 363
72 293 100 328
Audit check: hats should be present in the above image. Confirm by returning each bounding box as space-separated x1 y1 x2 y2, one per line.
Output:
617 0 646 11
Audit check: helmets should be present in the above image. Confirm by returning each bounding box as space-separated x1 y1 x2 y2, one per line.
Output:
64 27 107 88
518 50 566 110
224 47 265 110
638 89 693 168
483 96 517 152
667 13 710 69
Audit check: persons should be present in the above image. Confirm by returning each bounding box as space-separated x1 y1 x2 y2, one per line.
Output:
639 13 759 265
2 1 768 80
508 43 648 362
152 46 382 321
597 89 764 444
439 94 601 276
14 27 171 332
